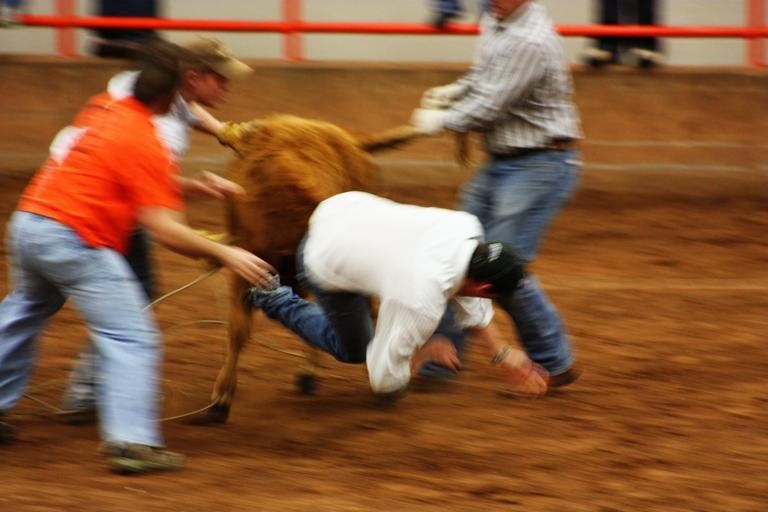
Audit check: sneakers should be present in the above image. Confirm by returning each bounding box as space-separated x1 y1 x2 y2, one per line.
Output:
103 443 189 473
243 272 271 312
53 409 97 425
539 366 581 386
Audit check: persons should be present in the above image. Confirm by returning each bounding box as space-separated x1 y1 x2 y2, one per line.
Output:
56 35 256 426
586 1 661 70
408 1 586 385
0 59 277 472
242 184 553 398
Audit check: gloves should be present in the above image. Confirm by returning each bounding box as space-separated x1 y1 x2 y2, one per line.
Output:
411 333 463 374
409 107 445 136
418 85 453 107
490 345 549 398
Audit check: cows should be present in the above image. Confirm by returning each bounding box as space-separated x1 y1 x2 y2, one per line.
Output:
205 108 475 421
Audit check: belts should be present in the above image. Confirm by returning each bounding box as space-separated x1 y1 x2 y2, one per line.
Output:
542 137 583 149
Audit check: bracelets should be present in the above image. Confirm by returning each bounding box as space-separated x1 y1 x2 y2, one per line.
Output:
492 343 511 366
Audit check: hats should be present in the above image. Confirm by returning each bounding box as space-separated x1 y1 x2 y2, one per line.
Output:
472 241 523 279
179 35 256 81
